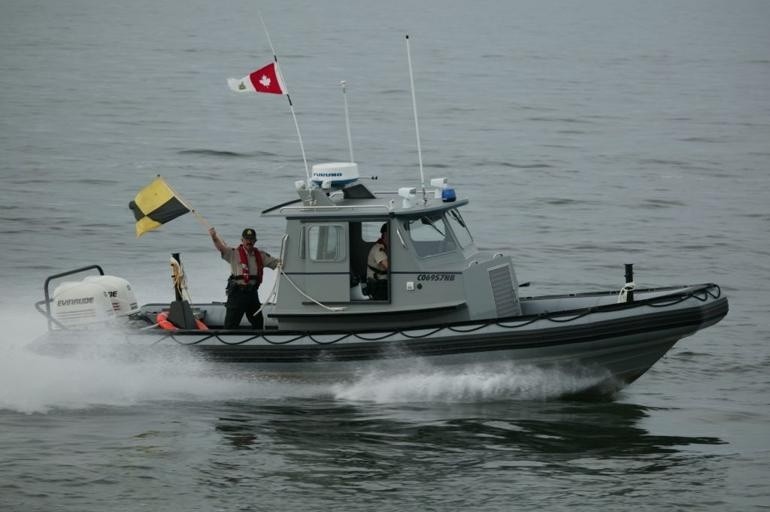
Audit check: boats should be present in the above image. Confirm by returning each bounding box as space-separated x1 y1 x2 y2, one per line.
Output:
26 162 728 398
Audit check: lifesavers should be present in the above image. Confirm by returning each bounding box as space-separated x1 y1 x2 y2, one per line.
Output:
156 311 208 330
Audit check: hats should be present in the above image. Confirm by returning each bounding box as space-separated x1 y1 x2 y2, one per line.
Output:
242 228 256 240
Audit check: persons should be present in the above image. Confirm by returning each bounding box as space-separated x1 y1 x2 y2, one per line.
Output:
366 223 389 301
208 226 279 329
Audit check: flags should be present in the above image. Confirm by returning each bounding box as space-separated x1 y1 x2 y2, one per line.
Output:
128 177 190 239
225 60 288 96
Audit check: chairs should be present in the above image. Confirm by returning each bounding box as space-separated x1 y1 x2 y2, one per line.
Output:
348 241 367 282
